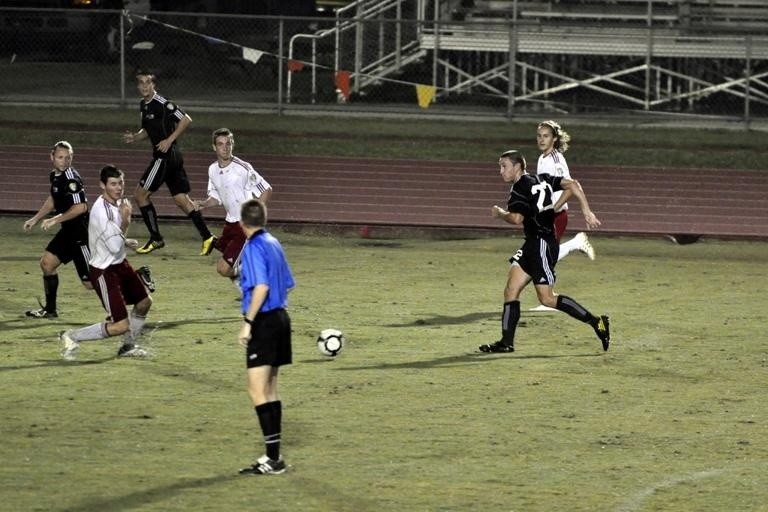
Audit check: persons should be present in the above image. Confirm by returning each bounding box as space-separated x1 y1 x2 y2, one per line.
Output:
479 149 611 354
58 165 152 363
186 129 272 299
122 69 219 256
236 202 294 478
22 141 154 319
527 120 595 312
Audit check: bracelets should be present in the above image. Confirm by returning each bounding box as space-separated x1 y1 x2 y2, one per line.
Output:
244 317 255 327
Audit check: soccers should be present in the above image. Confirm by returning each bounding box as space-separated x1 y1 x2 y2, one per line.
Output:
317 329 344 357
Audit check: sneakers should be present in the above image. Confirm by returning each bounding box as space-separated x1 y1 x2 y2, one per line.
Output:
22 308 59 321
200 235 217 257
595 315 611 352
116 346 154 359
238 454 287 477
57 329 78 363
136 237 166 254
478 341 515 354
135 266 155 293
529 304 560 313
576 231 595 261
